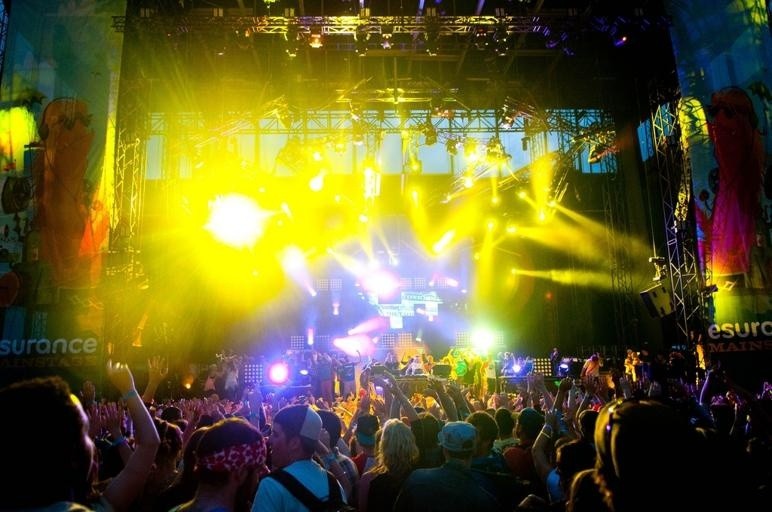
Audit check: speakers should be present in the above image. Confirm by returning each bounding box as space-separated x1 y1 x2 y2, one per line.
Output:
640 283 676 320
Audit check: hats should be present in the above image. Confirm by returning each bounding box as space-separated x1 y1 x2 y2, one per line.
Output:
436 421 477 452
273 404 323 440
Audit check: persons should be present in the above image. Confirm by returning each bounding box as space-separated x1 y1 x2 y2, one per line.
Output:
0 348 772 510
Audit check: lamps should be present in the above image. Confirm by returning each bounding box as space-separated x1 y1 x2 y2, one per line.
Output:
233 0 517 57
280 102 511 171
